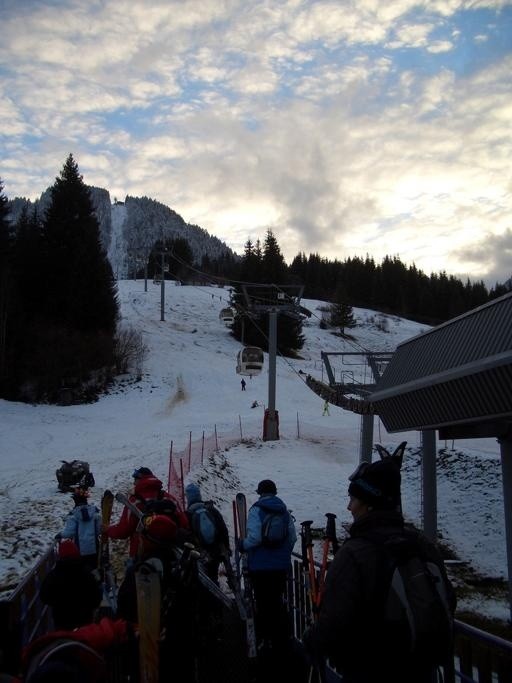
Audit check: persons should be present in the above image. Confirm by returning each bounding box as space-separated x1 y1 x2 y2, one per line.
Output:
301 455 457 683
322 400 331 416
238 479 298 648
241 378 247 391
21 461 232 683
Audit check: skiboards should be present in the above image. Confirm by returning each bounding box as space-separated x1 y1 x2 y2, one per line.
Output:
233 494 259 659
223 557 248 620
115 491 232 609
99 490 113 579
136 558 163 683
374 442 408 515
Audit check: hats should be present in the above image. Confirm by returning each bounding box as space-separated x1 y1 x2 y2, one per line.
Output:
255 479 277 497
185 484 201 501
132 466 152 476
347 456 403 504
143 514 183 537
54 535 81 560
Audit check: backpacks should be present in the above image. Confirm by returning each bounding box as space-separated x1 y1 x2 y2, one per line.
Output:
253 500 289 550
131 489 177 514
191 507 219 549
